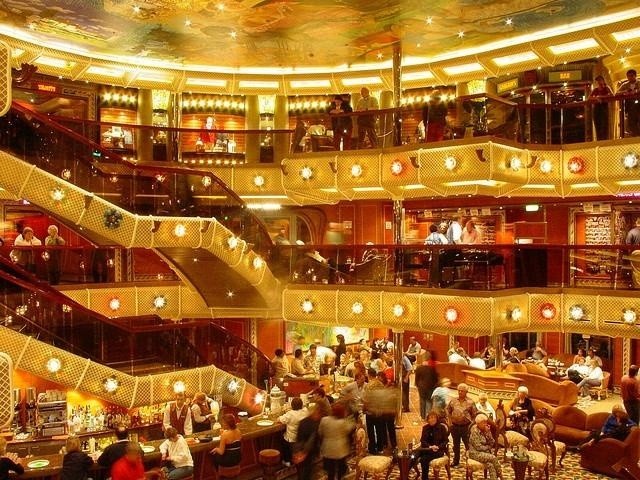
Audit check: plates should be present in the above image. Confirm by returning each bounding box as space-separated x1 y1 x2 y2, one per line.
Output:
28 459 50 468
257 420 273 427
88 450 103 461
141 445 155 453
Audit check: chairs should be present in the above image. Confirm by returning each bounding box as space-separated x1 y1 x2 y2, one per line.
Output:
391 248 505 290
351 397 640 480
282 339 609 404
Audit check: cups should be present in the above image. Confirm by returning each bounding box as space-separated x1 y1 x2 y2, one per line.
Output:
32 432 37 438
44 389 66 401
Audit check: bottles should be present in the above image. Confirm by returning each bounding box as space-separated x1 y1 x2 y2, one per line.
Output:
68 403 166 432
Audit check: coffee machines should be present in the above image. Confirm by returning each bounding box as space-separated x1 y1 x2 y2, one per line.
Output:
35 400 68 437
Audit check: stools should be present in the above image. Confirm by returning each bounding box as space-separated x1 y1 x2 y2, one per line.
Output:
258 448 281 479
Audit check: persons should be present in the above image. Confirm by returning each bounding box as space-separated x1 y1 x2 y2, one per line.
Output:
60 435 94 479
616 69 638 139
291 120 306 146
266 333 640 480
110 450 145 480
0 455 25 479
198 116 218 145
97 424 145 480
354 87 381 150
423 213 484 262
422 89 448 143
90 244 109 282
327 95 353 150
43 225 66 286
625 219 640 248
12 398 32 428
586 76 613 139
158 390 242 479
271 227 290 245
9 226 41 274
